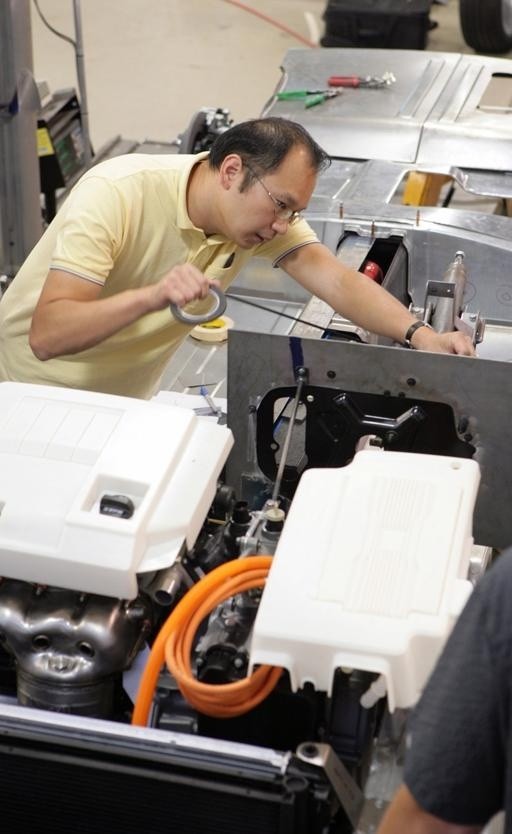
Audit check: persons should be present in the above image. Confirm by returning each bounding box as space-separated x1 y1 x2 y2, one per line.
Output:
0 117 474 400
375 546 512 834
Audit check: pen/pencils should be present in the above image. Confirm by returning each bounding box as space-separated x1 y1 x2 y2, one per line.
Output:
200 385 218 414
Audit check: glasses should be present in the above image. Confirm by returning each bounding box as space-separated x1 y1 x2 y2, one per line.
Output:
242 163 304 226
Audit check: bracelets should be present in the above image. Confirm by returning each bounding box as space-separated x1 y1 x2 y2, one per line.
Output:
404 320 432 349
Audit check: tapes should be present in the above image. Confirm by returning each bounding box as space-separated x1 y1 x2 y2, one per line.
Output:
188 315 236 345
166 283 223 329
